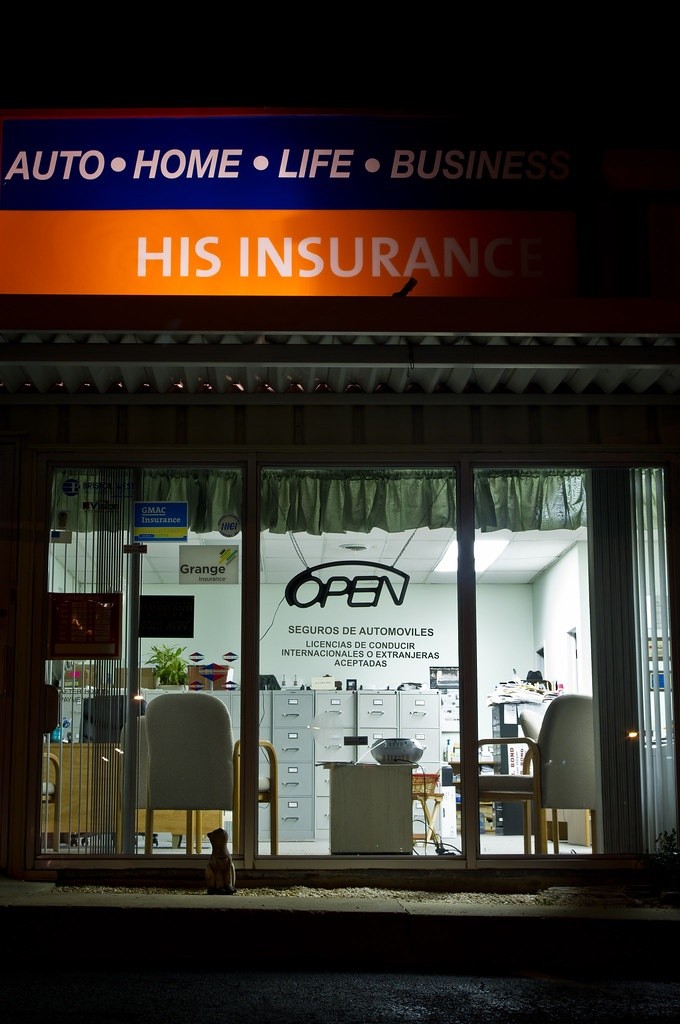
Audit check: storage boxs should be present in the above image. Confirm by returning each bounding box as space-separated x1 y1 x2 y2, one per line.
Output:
75 665 105 686
187 665 235 690
114 668 155 689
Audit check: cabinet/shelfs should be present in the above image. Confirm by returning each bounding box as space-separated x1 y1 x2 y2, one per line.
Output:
212 689 565 856
450 762 495 835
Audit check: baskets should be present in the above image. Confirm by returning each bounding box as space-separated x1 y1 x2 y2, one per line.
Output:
412 773 440 794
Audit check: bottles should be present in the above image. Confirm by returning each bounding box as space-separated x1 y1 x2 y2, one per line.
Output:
558 684 565 697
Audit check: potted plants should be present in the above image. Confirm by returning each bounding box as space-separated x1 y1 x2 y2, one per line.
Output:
143 642 189 694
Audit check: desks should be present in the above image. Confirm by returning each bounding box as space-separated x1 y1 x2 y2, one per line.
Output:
323 763 419 856
43 743 121 846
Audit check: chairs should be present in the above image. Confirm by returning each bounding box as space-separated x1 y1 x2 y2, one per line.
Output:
145 694 280 855
117 716 202 854
42 752 61 852
478 696 595 856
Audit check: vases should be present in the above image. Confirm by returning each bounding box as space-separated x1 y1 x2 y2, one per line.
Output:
371 738 424 763
